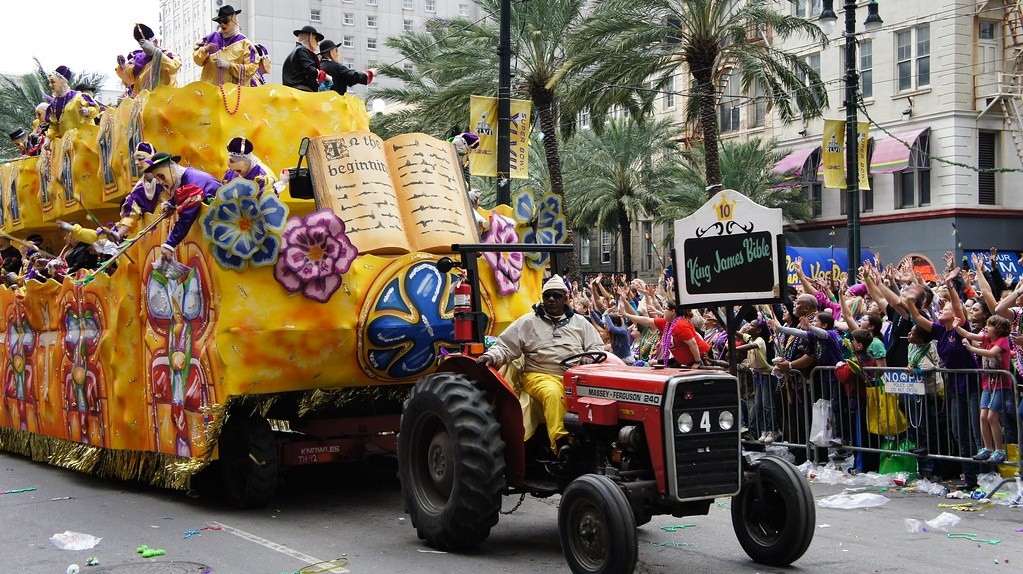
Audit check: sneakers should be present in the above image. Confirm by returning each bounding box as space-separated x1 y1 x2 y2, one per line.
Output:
987 449 1006 464
973 447 994 459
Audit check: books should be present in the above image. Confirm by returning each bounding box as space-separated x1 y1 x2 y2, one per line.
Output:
306 131 480 253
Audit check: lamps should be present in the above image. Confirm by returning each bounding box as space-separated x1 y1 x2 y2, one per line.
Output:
902 106 914 122
797 128 808 138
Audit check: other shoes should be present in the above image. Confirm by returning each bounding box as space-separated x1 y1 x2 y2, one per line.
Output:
957 484 974 491
759 431 771 442
828 437 843 445
764 429 783 443
557 445 573 462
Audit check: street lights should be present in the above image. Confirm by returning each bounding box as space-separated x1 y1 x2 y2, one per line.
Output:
816 0 884 286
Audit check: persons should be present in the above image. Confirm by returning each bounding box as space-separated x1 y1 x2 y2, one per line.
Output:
223 137 278 199
0 218 120 284
10 66 106 157
107 141 223 259
282 26 377 95
473 274 605 457
193 5 271 87
568 247 1023 462
115 23 181 103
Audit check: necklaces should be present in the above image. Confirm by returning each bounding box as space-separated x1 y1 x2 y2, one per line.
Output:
661 317 682 366
1011 313 1023 377
788 315 814 362
639 330 657 362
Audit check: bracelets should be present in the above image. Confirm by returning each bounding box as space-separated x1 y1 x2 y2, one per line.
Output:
948 284 954 290
1015 291 1021 296
789 362 792 368
825 288 828 289
908 300 911 302
865 276 870 280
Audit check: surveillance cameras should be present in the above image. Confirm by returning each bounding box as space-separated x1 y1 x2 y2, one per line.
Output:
799 129 806 134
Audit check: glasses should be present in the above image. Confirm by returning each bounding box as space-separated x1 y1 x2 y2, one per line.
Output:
542 292 565 299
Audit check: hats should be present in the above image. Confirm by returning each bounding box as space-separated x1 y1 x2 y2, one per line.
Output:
75 84 95 92
293 26 324 41
542 274 569 293
254 44 268 58
134 142 156 159
145 152 181 174
227 137 253 158
9 129 25 140
317 40 342 56
54 66 71 82
212 5 242 21
134 24 154 41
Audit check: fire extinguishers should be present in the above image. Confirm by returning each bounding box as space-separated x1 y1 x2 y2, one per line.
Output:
453 273 472 341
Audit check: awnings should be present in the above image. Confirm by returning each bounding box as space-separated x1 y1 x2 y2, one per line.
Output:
818 137 873 181
771 146 821 186
869 128 931 174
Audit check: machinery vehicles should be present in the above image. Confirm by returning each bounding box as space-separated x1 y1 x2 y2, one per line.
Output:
205 242 816 574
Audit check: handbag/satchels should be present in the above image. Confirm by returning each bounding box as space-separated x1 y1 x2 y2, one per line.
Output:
865 370 907 435
809 399 836 447
